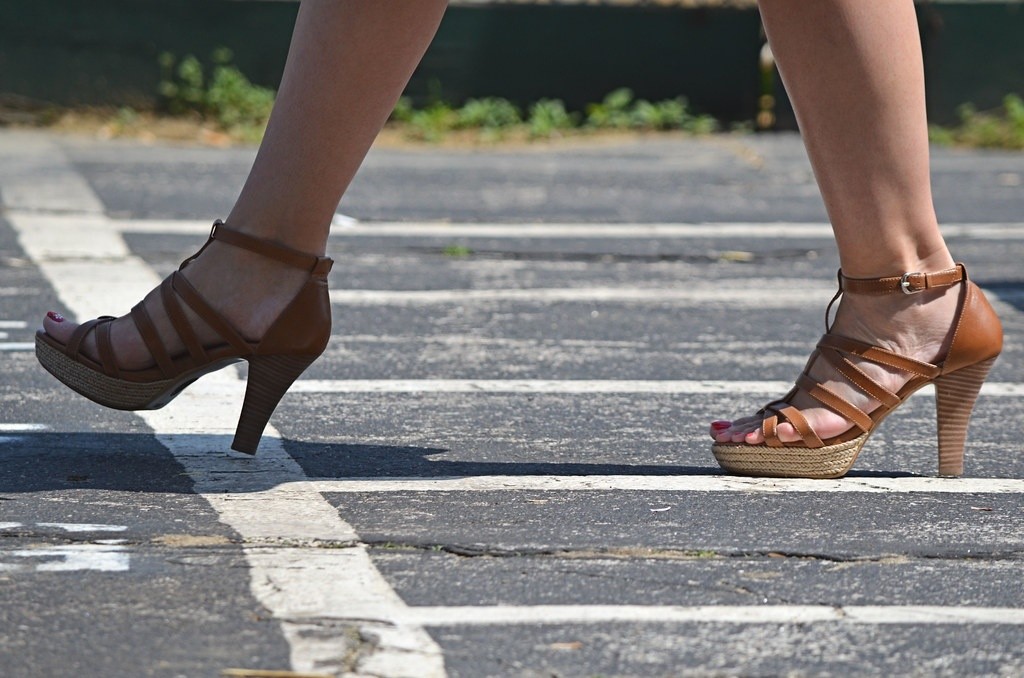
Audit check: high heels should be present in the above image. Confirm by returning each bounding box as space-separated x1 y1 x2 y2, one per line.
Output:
33 219 334 456
711 263 1003 480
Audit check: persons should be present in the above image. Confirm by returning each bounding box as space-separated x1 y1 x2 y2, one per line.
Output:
33 0 1002 481
